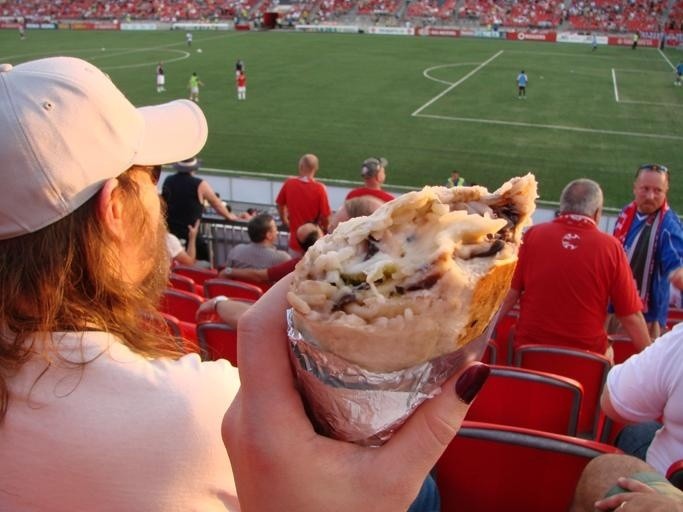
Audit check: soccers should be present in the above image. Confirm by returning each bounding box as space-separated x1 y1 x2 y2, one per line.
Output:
196 48 202 53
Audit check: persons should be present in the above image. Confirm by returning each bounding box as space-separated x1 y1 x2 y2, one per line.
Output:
674 61 683 85
189 72 204 102
602 322 682 475
0 55 242 511
345 158 395 204
502 177 649 362
613 164 682 336
235 59 243 80
221 273 682 511
516 69 528 100
0 0 683 52
447 170 467 187
159 154 384 327
237 72 246 100
157 59 167 92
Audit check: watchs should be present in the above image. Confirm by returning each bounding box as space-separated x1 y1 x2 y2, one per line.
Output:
214 295 228 311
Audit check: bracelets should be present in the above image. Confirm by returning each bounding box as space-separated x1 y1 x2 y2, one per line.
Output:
226 267 232 274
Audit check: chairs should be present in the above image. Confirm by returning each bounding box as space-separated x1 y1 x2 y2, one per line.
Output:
136 259 683 512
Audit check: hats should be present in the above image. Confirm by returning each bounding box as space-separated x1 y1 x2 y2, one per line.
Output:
174 158 200 172
0 56 209 240
361 156 389 178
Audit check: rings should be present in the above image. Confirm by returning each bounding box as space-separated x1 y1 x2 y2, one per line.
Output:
621 501 628 510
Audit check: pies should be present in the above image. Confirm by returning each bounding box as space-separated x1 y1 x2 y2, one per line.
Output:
287 171 538 371
